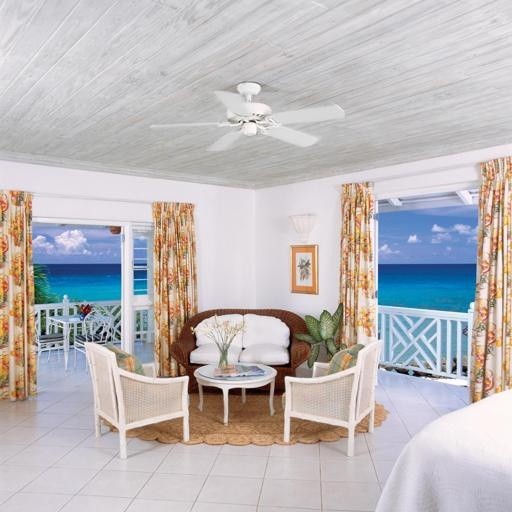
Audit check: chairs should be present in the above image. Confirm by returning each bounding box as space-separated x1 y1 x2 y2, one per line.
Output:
283 338 383 457
34 308 65 364
84 342 190 459
74 310 113 375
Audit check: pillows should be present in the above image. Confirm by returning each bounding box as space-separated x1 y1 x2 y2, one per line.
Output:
328 344 365 375
105 342 146 376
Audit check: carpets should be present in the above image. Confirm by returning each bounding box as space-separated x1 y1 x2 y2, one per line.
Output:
99 394 389 445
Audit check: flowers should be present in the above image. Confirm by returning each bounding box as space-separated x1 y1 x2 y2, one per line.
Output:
189 313 246 365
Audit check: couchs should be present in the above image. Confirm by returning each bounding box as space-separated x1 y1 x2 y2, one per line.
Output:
169 309 311 394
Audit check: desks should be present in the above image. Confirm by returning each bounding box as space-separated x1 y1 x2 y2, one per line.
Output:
46 314 114 371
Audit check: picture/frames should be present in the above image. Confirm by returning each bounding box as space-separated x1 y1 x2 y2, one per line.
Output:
290 244 320 295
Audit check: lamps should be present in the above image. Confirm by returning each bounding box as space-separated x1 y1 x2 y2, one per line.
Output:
226 80 272 136
290 213 316 242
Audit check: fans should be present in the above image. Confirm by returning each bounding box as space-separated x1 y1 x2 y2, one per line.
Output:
150 82 346 153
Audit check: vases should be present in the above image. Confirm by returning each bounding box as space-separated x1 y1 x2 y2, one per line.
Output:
217 344 230 370
